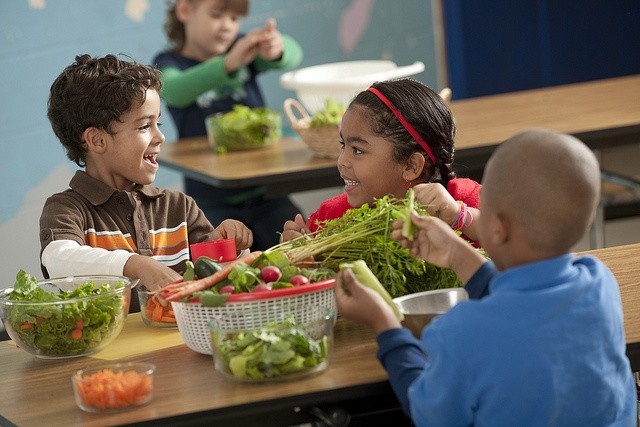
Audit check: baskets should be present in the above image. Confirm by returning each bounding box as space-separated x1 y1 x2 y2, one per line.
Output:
284 87 452 157
159 269 337 356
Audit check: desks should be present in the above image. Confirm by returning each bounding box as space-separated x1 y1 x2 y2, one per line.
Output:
156 74 639 248
0 236 640 427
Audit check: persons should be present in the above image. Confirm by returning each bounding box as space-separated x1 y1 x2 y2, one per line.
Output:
334 128 636 426
283 79 484 248
151 1 305 144
39 53 252 290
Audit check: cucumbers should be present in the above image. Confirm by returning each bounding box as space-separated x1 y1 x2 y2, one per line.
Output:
194 256 222 279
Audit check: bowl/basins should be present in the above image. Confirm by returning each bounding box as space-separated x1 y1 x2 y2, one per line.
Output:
70 363 157 414
209 304 334 385
281 60 426 114
392 287 469 340
204 110 281 152
5 275 140 359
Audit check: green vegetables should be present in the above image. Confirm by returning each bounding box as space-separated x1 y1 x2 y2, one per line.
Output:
265 192 491 299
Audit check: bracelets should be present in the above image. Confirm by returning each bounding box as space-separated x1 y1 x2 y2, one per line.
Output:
455 197 471 235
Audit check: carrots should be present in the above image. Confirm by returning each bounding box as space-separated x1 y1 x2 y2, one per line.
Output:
76 369 153 410
143 291 176 324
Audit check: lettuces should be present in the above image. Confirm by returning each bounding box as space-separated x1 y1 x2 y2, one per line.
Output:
211 102 281 155
308 97 345 130
1 269 127 361
